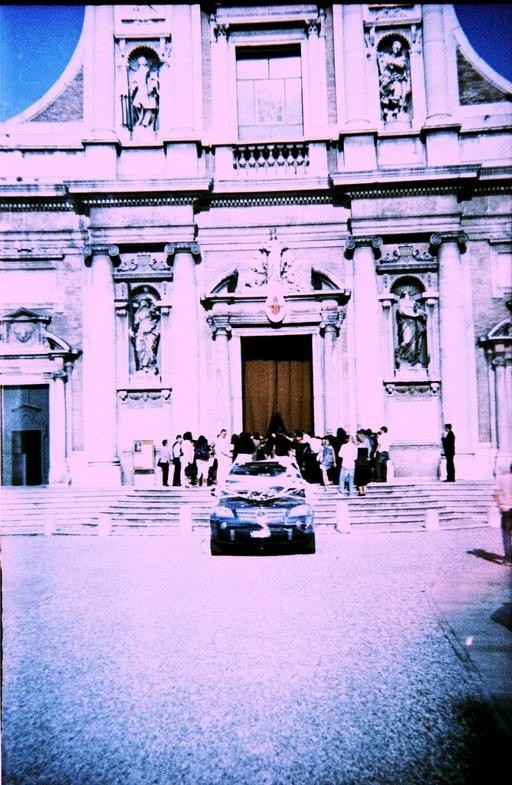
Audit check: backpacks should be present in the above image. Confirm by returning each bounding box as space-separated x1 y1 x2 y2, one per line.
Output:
318 445 334 467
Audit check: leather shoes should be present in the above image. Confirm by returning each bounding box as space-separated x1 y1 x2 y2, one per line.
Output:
444 480 455 482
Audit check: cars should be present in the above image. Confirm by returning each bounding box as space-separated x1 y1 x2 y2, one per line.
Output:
210 453 316 555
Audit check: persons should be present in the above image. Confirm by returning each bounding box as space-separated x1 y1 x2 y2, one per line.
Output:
396 289 427 365
132 295 160 370
158 427 392 497
441 424 455 482
377 41 412 112
131 56 159 127
494 462 512 566
